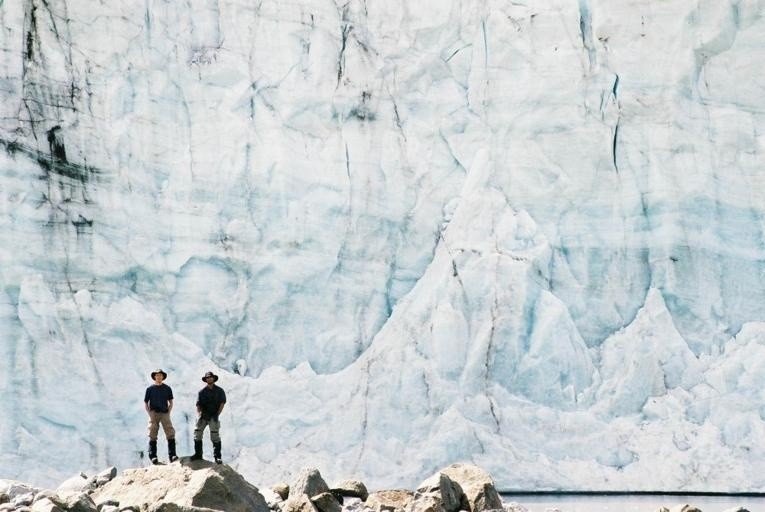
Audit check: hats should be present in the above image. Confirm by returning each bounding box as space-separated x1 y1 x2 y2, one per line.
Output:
202 372 218 382
151 368 167 380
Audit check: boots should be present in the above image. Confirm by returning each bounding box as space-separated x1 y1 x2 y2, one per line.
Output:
191 440 202 461
168 438 178 462
213 441 222 464
148 440 157 463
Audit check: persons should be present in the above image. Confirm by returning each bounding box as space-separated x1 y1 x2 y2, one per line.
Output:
144 370 178 465
189 371 227 465
